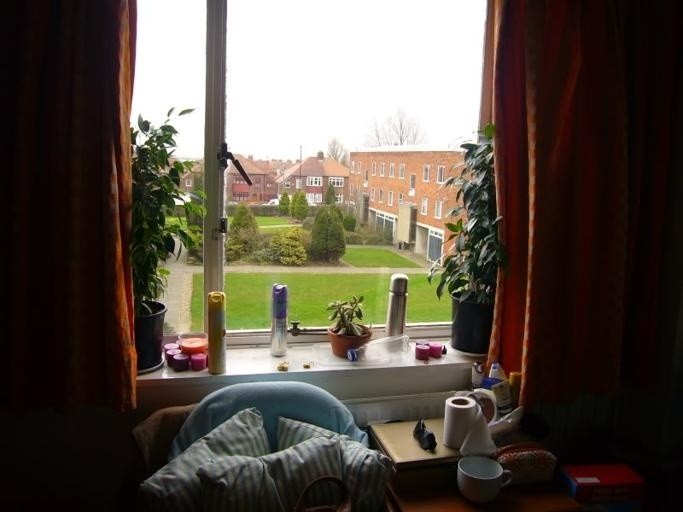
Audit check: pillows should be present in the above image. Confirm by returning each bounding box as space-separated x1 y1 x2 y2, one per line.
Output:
138 405 398 512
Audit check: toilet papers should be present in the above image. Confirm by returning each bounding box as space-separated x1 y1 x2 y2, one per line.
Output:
443 395 497 458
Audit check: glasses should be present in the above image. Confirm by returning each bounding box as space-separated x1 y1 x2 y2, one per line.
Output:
414 418 436 452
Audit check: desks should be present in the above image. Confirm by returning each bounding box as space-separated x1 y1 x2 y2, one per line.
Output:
381 458 610 512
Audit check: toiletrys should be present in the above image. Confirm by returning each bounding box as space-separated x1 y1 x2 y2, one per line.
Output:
489 362 509 381
471 361 486 391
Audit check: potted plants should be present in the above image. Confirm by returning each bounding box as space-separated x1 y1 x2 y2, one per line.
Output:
426 121 513 361
324 295 374 359
124 105 208 377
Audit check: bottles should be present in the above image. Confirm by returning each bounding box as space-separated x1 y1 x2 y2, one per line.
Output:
206 291 227 375
385 273 409 337
347 334 410 363
269 283 288 357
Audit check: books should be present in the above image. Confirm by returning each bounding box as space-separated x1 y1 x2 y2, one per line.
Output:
561 461 646 499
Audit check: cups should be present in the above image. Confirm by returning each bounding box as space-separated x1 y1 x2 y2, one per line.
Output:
457 456 513 503
